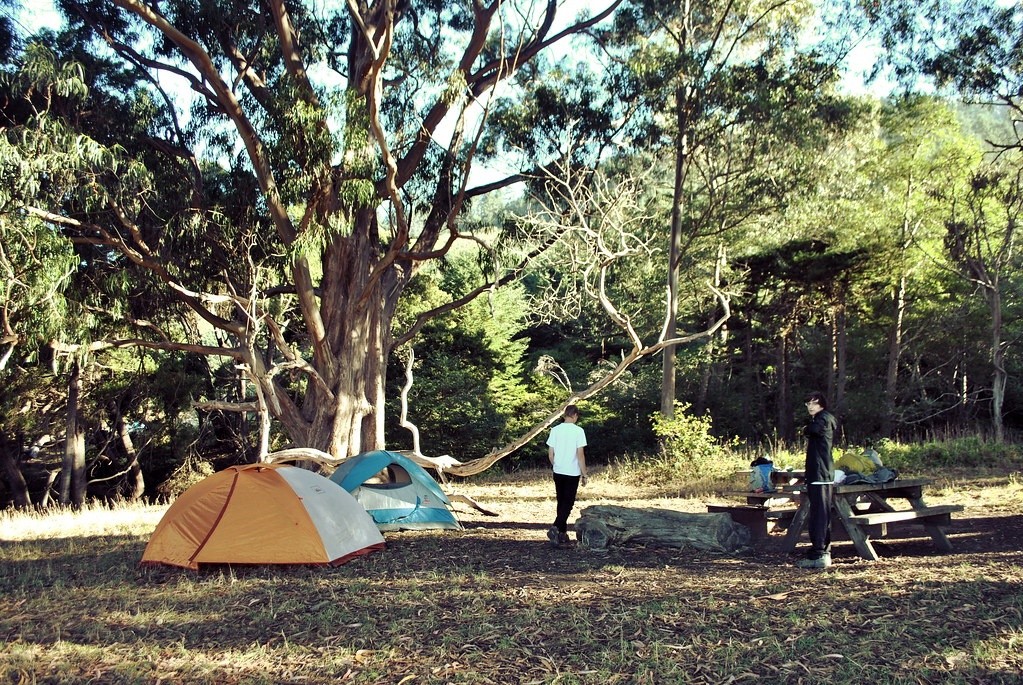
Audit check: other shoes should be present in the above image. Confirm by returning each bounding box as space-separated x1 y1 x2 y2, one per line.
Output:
797 552 832 568
547 526 570 550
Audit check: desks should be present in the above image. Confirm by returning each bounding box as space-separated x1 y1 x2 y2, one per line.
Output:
725 469 954 559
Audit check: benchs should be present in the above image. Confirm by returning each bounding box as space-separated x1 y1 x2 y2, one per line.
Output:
707 505 797 537
849 505 964 537
730 491 799 499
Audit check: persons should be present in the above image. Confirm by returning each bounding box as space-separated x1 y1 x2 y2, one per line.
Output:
795 390 838 569
545 405 588 549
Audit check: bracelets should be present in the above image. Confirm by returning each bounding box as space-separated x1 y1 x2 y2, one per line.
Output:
582 476 586 478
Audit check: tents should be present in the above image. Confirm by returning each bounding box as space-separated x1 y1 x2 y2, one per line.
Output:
328 450 466 532
136 462 386 571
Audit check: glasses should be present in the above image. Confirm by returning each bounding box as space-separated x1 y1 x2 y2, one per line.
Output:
560 413 574 420
805 402 818 406
